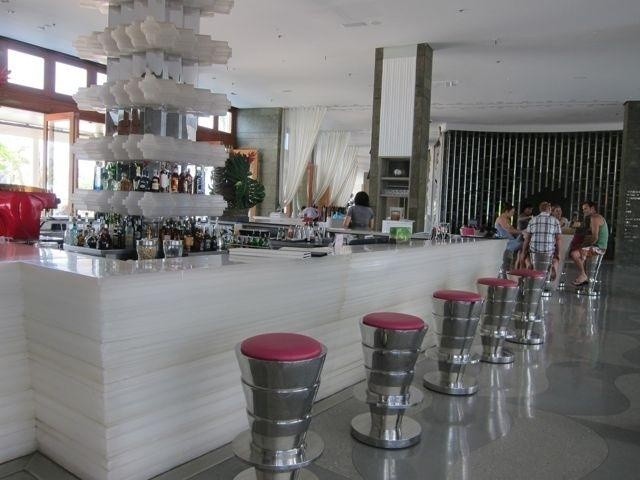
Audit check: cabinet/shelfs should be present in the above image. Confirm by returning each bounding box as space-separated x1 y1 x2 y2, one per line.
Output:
373 155 413 234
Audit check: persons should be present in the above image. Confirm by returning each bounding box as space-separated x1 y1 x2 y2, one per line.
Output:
493 199 610 288
301 205 321 222
342 191 375 229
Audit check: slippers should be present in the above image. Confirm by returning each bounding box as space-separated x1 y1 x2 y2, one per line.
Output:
571 279 589 286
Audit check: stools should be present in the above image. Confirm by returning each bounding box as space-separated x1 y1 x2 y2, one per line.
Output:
530 253 605 296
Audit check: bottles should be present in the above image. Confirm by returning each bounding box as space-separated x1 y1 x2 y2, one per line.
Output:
63 213 272 259
91 160 205 194
277 225 325 246
430 225 451 240
